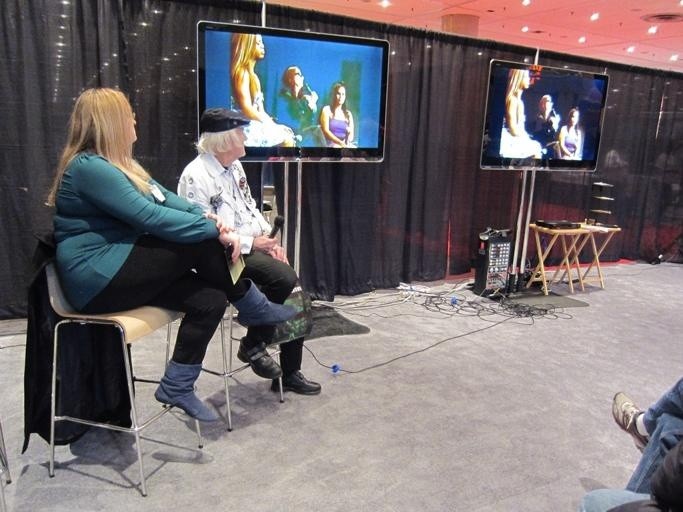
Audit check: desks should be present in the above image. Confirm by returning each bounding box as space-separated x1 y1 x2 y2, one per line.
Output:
527 222 622 296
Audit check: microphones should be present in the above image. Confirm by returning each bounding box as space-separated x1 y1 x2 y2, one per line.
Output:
266 214 284 238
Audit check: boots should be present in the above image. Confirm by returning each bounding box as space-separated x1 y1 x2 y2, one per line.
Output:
155 361 216 421
231 279 297 327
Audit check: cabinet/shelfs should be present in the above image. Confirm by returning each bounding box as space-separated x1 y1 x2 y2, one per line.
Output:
589 181 615 227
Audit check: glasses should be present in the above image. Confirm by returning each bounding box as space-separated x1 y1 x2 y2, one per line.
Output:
295 73 303 76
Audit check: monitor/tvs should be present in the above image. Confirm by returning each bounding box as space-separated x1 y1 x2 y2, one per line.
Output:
197 20 390 162
479 59 609 171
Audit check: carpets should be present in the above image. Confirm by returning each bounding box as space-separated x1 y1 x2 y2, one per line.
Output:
234 304 371 344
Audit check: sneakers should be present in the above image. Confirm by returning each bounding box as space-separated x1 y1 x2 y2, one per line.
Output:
271 372 321 394
612 393 650 453
237 338 283 379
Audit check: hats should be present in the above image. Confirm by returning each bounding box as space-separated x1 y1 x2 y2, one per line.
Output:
200 109 250 132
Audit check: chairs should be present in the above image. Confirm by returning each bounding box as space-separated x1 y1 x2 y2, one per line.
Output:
163 210 284 432
0 425 12 511
44 261 203 497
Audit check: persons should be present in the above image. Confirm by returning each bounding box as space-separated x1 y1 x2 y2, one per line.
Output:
578 439 683 512
229 33 295 149
46 87 296 420
535 92 563 162
177 108 321 393
281 66 317 146
559 107 582 162
498 69 541 161
612 376 682 491
318 80 355 150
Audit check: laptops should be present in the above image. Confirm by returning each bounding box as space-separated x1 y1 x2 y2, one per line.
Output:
536 219 581 229
